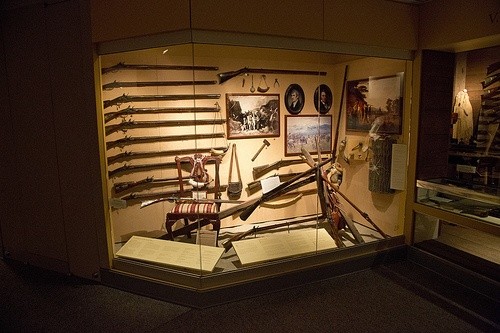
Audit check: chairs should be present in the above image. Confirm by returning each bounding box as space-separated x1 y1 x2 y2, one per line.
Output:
166 153 224 247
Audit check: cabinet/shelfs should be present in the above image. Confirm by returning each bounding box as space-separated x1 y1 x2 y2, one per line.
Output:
414 178 500 237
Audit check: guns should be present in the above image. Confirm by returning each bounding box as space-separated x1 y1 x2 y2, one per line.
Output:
247 157 330 191
101 61 230 211
139 198 244 210
217 213 324 253
216 66 328 84
239 155 338 222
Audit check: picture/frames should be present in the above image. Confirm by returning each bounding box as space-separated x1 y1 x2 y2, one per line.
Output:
225 93 280 139
315 84 333 114
284 114 332 157
345 71 405 135
285 84 305 115
